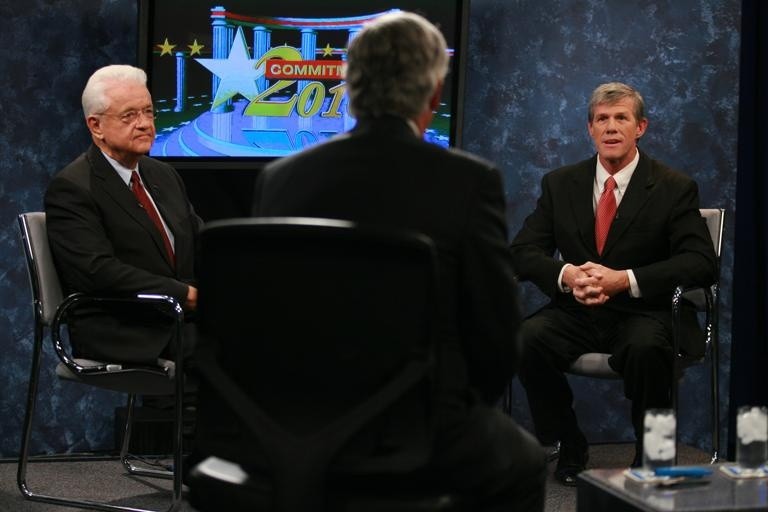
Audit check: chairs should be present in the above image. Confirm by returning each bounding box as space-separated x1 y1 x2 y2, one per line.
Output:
183 220 445 510
501 205 724 467
14 209 190 512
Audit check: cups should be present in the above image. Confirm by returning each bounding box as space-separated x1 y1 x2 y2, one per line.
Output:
641 408 679 474
736 404 768 469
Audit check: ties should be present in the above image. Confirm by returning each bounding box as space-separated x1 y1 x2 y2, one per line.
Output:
595 176 617 256
130 171 175 272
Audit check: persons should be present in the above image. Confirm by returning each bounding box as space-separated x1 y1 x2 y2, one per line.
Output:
511 82 722 488
253 11 548 511
43 64 206 377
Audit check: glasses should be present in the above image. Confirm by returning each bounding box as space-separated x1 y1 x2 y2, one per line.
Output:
95 107 160 123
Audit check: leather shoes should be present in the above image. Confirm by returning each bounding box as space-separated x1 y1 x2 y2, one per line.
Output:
561 441 587 486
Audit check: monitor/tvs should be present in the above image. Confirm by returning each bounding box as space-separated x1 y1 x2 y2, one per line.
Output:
136 0 472 172
